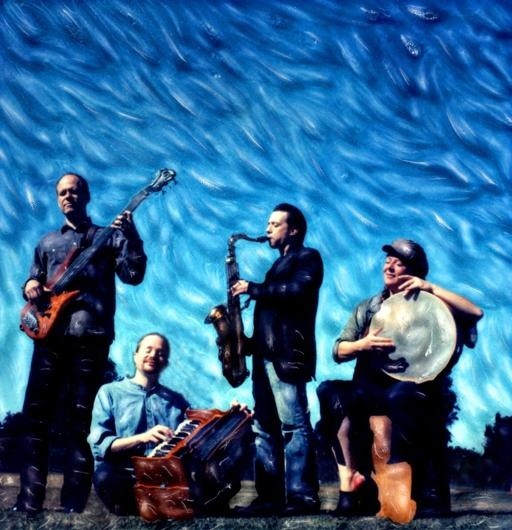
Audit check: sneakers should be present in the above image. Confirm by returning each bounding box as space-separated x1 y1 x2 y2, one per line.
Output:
235 499 319 515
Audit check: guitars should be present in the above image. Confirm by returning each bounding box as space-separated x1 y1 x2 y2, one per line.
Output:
20 168 177 339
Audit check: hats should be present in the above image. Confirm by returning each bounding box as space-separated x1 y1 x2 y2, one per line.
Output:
383 240 428 275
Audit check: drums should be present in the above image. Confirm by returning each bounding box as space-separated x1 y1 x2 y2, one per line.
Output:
369 290 463 385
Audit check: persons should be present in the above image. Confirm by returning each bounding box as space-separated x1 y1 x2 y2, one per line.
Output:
4 172 148 515
229 202 325 516
85 332 255 516
314 238 484 516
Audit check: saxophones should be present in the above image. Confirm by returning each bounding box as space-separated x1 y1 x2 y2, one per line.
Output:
203 233 271 388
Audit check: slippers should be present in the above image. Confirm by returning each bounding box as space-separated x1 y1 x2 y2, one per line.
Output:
334 489 358 516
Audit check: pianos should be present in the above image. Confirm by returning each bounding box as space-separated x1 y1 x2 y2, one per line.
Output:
136 408 256 519
